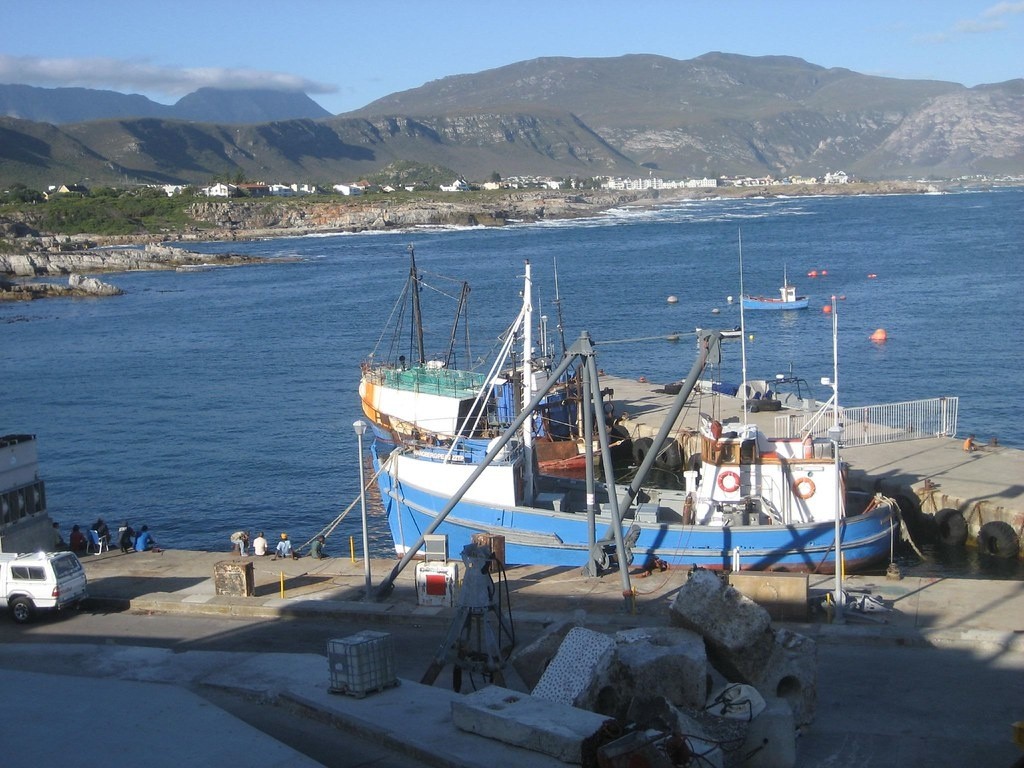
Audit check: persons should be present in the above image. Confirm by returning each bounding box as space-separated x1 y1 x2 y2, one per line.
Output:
253 532 273 556
270 532 298 561
964 434 977 453
53 517 158 554
230 530 251 557
311 535 330 559
599 418 633 476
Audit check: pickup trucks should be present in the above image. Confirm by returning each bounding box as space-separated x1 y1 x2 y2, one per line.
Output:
0 551 89 623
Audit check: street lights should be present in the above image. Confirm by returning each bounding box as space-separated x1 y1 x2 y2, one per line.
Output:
828 425 844 625
352 420 370 598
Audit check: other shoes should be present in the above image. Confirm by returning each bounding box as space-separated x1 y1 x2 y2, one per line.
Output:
121 549 128 554
242 553 249 557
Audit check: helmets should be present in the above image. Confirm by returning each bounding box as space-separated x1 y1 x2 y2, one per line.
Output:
281 533 287 539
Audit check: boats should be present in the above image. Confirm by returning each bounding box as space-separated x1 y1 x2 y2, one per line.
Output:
371 224 898 576
741 258 809 311
357 240 495 443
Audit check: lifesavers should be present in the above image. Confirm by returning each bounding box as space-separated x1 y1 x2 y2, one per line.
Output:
792 477 817 500
717 471 742 494
631 435 686 472
